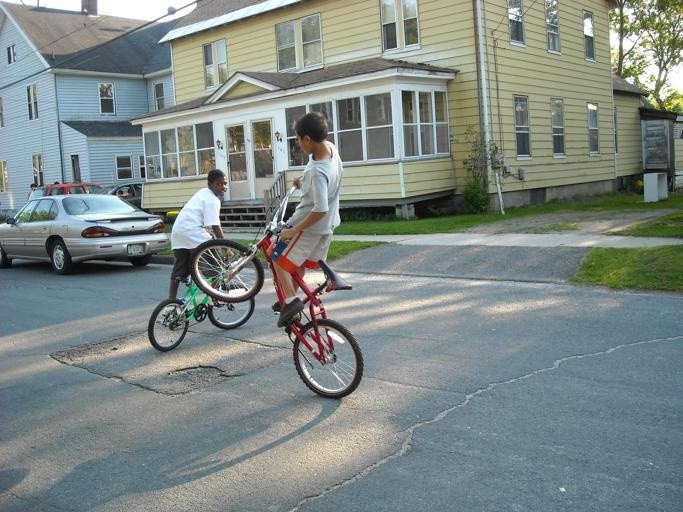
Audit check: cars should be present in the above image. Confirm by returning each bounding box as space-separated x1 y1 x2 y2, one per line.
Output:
29 183 142 208
0 194 168 275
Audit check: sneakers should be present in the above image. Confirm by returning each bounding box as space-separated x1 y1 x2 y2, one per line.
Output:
162 304 176 318
277 297 305 328
272 300 280 311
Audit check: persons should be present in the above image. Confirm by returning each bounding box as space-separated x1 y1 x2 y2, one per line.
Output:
271 111 344 328
29 183 44 200
162 169 235 317
